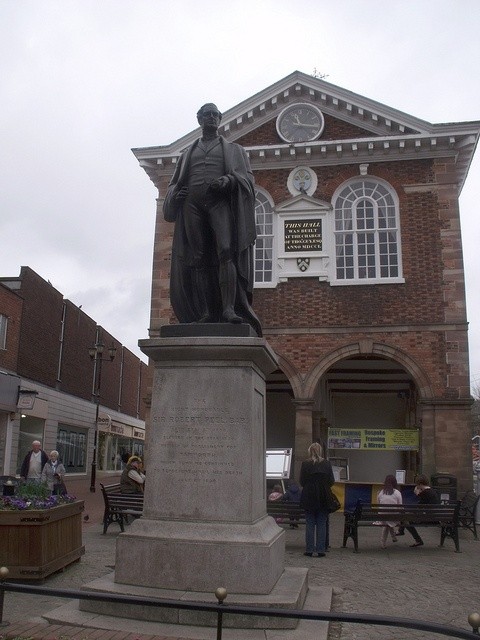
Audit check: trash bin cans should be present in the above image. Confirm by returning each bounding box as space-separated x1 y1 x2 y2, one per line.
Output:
431 472 457 505
0 476 18 496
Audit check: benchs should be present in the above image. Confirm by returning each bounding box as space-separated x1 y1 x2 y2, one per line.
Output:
101 483 130 524
443 489 480 540
99 483 144 535
266 499 331 553
343 500 463 552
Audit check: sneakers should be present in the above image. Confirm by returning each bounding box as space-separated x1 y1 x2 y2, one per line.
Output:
303 552 313 556
318 553 325 557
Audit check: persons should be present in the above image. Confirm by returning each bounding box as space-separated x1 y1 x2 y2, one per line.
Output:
299 443 341 557
268 484 284 523
162 103 264 326
41 450 68 496
20 440 49 483
372 474 403 549
274 479 302 529
119 455 146 518
394 476 439 548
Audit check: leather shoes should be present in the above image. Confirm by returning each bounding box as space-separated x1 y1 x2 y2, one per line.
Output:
410 541 424 547
394 531 405 536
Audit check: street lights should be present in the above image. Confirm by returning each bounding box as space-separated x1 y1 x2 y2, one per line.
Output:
88 338 117 492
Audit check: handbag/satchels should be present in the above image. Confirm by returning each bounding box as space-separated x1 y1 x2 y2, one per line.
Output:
328 487 341 513
53 479 67 495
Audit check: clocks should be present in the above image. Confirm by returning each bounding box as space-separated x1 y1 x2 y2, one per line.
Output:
276 102 325 143
